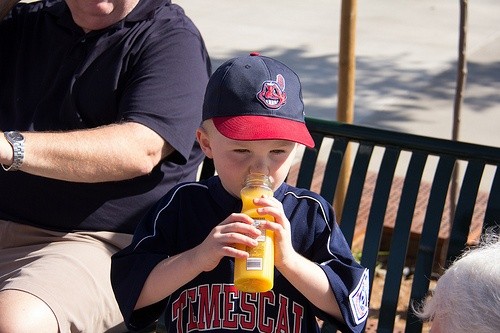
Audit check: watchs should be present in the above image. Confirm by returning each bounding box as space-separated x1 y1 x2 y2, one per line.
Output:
1 129 25 172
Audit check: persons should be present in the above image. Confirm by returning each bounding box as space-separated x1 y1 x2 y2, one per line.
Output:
108 50 372 333
0 0 212 333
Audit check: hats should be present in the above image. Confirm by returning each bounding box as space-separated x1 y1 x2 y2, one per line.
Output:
201 51 316 149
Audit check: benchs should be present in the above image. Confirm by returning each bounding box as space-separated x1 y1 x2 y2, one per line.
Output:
191 115 500 332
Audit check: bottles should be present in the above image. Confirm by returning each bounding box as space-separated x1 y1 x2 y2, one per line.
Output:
234 173 277 294
